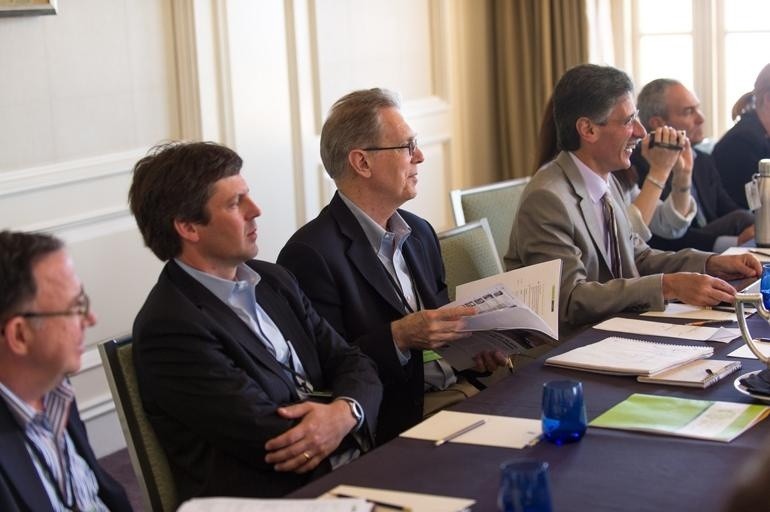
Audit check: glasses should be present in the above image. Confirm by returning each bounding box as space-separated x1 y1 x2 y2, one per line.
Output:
2 288 89 337
362 137 417 157
608 110 640 126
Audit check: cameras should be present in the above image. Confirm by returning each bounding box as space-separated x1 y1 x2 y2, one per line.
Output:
649 131 686 150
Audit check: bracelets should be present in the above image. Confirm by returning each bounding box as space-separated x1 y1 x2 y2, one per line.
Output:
646 174 665 190
670 185 691 193
348 400 362 421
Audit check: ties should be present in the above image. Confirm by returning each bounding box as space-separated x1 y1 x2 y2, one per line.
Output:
600 192 620 278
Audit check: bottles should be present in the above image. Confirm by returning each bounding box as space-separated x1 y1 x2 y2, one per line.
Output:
751 158 770 249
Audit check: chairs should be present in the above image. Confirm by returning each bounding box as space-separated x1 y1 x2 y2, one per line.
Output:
447 174 533 274
95 330 187 512
432 214 505 302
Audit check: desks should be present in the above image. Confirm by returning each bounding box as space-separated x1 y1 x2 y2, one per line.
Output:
273 269 770 511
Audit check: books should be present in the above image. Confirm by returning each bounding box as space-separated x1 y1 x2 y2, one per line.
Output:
636 358 742 389
588 393 769 443
430 258 564 372
544 336 714 378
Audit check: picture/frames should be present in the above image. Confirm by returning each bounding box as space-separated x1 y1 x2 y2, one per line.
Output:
0 0 59 17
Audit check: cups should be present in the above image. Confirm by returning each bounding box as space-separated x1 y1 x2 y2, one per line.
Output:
537 378 588 448
759 263 770 312
494 460 553 512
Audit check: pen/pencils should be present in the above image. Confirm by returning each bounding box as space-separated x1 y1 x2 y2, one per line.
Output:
328 491 411 512
688 320 734 326
434 419 487 446
528 434 545 447
749 251 770 257
756 338 770 342
712 307 754 314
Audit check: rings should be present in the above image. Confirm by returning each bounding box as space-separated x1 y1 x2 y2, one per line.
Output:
304 452 312 460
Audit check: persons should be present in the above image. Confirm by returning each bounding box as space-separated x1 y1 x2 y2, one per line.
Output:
710 63 770 210
503 62 764 331
531 88 698 242
0 229 135 511
126 136 385 505
636 77 755 253
274 87 510 445
